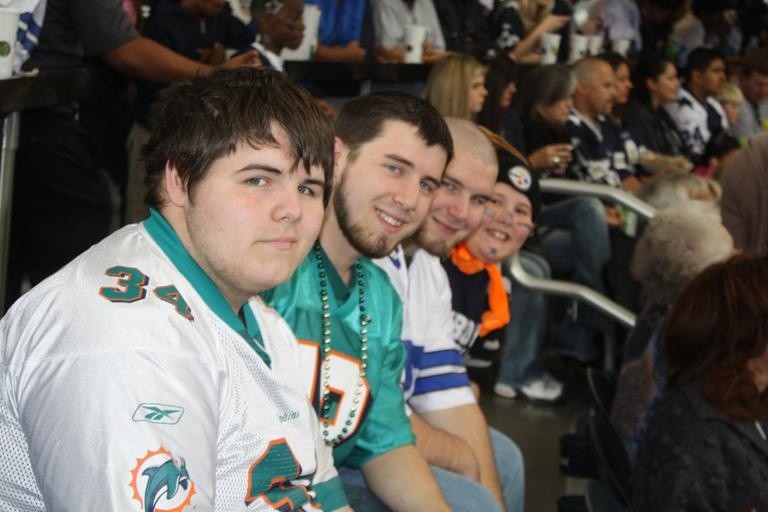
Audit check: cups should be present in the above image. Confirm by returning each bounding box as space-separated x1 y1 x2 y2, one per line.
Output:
538 30 630 66
402 24 428 64
0 7 20 80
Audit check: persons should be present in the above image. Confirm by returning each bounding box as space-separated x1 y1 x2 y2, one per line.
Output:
371 117 524 510
0 67 354 511
437 138 541 403
610 131 768 512
505 1 767 399
258 90 454 510
2 3 559 400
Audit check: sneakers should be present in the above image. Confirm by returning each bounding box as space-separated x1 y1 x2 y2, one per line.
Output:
460 338 501 369
491 372 563 401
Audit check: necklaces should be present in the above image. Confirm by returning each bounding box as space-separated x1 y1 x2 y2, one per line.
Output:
313 237 367 447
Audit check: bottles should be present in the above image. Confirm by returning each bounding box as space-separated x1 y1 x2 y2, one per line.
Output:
623 186 639 237
